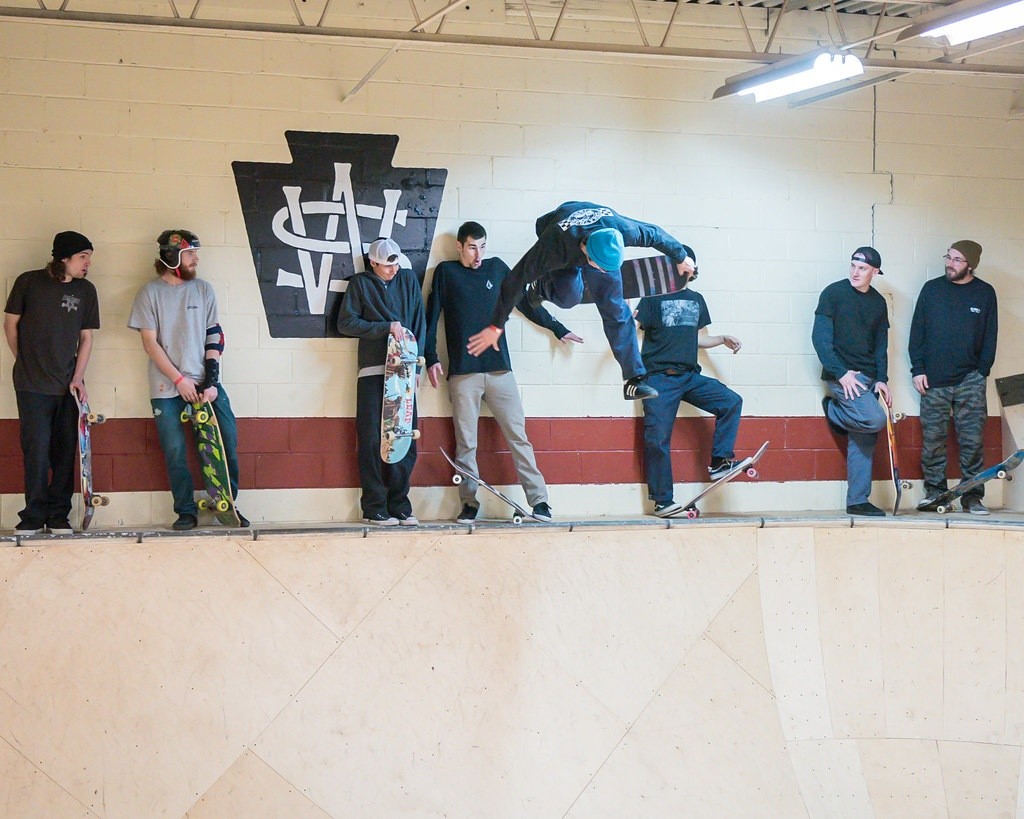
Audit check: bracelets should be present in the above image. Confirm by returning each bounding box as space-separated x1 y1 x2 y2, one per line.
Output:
174 375 185 385
489 324 506 334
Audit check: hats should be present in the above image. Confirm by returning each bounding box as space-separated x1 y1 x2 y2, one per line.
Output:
157 228 201 270
680 244 696 265
851 247 884 275
368 238 400 264
951 240 983 270
51 230 94 261
586 226 624 272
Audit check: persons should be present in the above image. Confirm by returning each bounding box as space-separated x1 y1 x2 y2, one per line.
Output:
127 227 252 529
425 220 586 526
907 240 999 516
811 247 893 518
465 197 696 401
624 243 751 518
336 237 427 527
3 230 101 535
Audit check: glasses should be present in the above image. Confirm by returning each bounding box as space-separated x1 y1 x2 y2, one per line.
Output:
943 254 966 265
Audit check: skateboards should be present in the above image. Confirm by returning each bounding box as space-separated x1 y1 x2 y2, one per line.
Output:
438 445 552 524
379 327 426 464
73 384 110 532
877 388 913 517
577 250 691 304
178 393 243 527
917 448 1024 513
658 439 772 519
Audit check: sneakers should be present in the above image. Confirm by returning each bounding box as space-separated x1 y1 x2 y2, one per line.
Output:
361 511 399 525
45 520 75 535
531 502 553 522
12 519 44 536
390 512 420 525
527 277 542 309
654 500 681 516
708 457 753 481
172 514 198 530
624 378 659 400
822 395 848 438
236 510 250 527
847 503 886 517
457 506 478 524
920 494 991 515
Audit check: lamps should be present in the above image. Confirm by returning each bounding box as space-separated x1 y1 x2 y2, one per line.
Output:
891 3 1024 46
709 44 865 103
